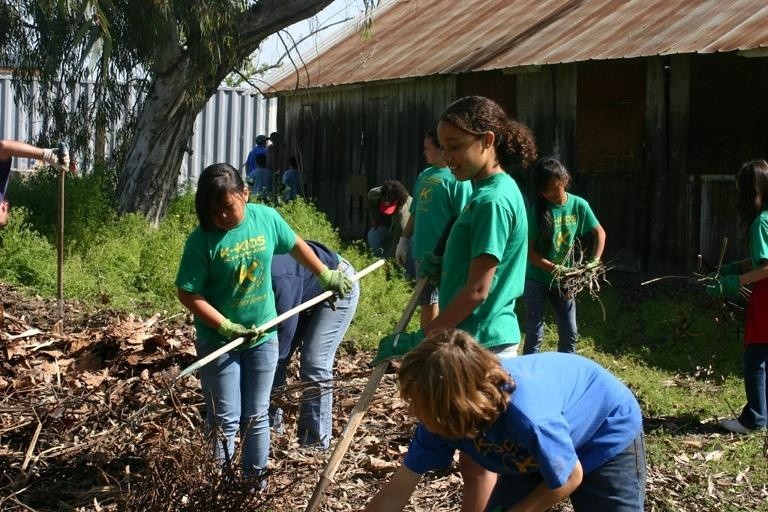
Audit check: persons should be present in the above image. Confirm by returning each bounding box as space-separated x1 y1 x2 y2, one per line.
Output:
435 96 534 512
395 131 474 328
247 132 301 203
368 179 415 278
173 160 354 487
0 137 70 228
523 158 603 356
719 156 766 434
265 238 362 451
352 329 647 510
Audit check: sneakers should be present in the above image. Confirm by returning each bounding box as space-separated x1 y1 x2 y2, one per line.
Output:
721 419 749 434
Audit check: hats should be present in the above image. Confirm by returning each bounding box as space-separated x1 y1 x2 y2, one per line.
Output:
379 196 399 215
256 135 269 143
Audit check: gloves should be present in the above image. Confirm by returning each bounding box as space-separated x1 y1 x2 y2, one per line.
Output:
317 265 353 303
413 248 441 286
585 256 600 269
369 329 424 374
705 261 740 298
217 318 265 349
396 237 408 267
551 264 575 288
